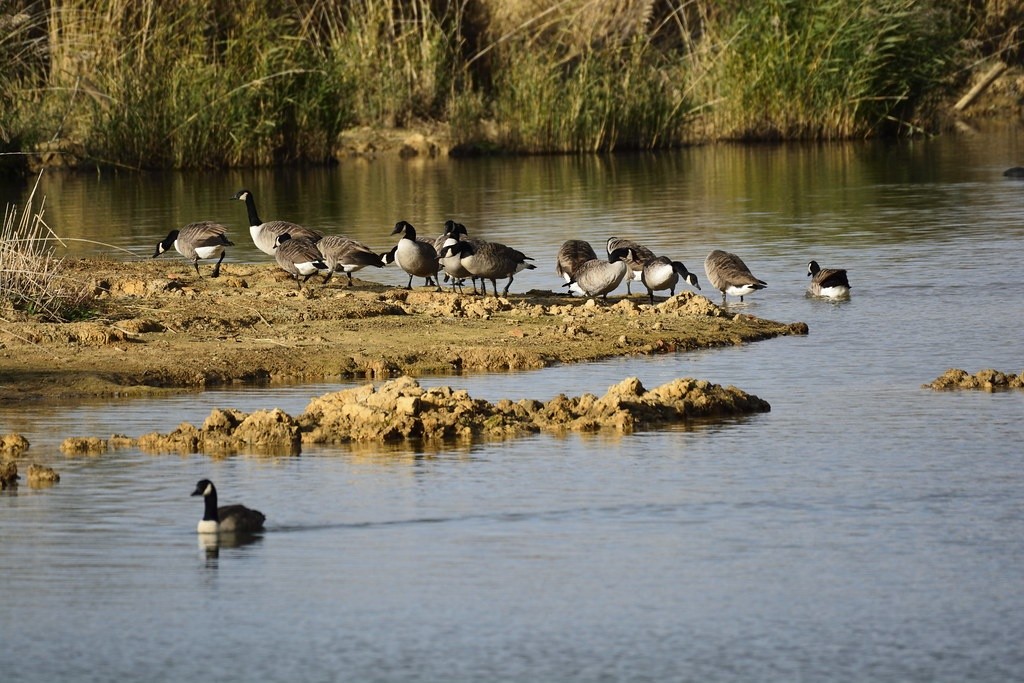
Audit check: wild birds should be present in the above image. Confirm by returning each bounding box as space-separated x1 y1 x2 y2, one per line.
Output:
378 220 538 299
189 479 266 534
562 247 641 306
555 240 598 295
704 249 768 302
273 233 329 291
606 236 656 295
303 235 387 287
641 256 701 305
152 220 235 278
229 189 323 258
807 260 853 298
